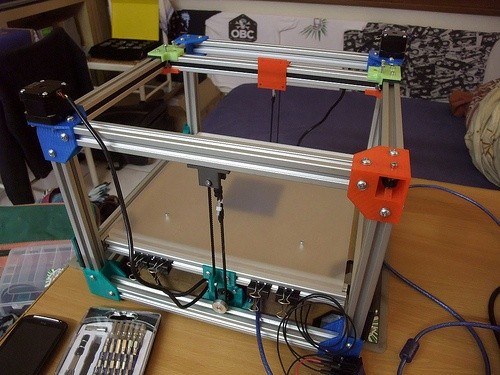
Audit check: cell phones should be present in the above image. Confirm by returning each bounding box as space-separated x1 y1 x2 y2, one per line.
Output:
0 314 67 375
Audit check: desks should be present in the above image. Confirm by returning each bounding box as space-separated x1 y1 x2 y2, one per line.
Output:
1 161 500 375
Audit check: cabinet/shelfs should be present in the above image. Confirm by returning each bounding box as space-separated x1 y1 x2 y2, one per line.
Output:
0 0 109 87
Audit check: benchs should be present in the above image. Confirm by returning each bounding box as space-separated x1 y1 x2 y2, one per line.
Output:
200 82 500 190
